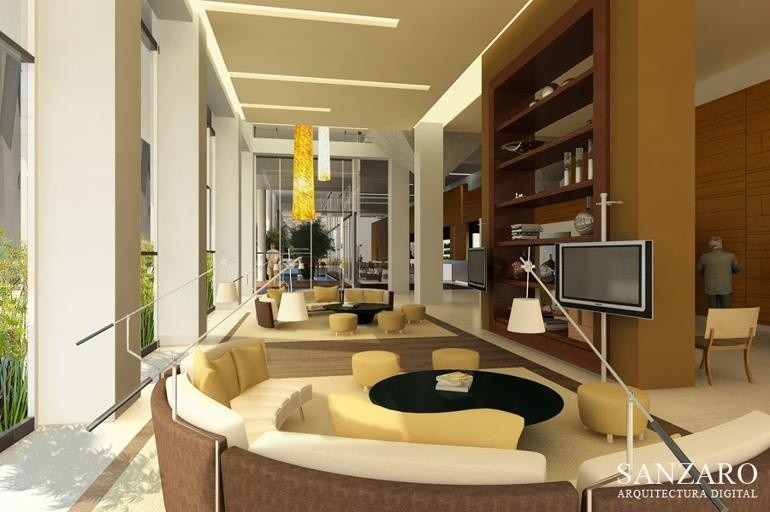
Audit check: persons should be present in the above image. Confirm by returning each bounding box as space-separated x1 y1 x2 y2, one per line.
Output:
266 241 279 280
698 235 741 308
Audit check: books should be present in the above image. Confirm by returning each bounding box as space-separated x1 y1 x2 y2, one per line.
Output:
435 371 474 393
545 321 568 332
510 223 543 240
342 302 359 308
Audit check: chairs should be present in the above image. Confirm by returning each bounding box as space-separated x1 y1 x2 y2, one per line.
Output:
694 305 760 387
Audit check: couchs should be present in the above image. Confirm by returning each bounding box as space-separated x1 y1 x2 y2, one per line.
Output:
254 288 394 330
151 339 769 512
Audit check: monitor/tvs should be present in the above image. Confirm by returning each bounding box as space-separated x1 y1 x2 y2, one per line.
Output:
555 240 653 320
468 247 488 292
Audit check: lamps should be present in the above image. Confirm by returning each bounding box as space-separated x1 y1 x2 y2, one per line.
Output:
290 124 331 222
76 244 310 431
506 247 732 510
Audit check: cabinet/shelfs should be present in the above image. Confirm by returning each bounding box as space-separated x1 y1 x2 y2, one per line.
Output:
489 0 610 377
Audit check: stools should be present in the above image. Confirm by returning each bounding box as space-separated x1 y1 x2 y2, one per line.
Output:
576 379 650 442
328 313 358 337
350 345 482 387
377 304 425 334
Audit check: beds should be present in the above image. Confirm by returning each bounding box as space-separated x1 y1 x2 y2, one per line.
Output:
324 301 392 325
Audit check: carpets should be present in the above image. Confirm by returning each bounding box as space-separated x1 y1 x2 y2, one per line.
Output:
275 366 670 481
231 307 457 340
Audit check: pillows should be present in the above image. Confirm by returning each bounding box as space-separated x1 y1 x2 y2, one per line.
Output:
194 342 269 410
313 285 339 304
268 287 281 303
